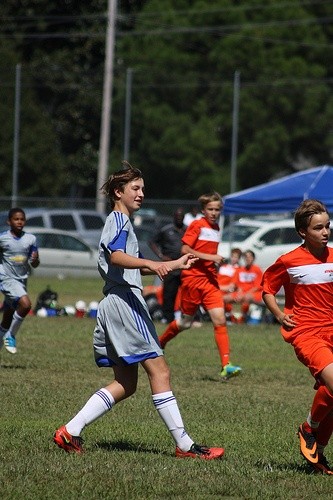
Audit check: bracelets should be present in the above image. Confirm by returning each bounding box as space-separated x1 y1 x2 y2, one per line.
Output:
159 254 162 258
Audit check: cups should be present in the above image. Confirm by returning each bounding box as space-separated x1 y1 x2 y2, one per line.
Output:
37 308 56 318
76 301 85 317
88 301 98 317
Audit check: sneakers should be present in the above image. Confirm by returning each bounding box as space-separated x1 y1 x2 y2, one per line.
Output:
308 448 333 475
2 333 17 354
298 421 318 464
53 426 84 453
177 443 224 459
220 362 241 380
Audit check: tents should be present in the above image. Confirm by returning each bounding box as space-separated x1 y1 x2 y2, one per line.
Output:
218 165 333 239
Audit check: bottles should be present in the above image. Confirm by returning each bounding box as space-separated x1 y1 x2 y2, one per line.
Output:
50 294 56 308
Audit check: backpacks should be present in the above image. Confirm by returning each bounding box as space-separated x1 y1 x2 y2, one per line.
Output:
34 285 58 315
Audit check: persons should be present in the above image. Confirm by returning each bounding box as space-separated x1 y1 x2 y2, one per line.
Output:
260 201 333 476
51 162 226 460
149 207 187 324
0 208 41 355
183 204 204 226
223 250 280 325
217 249 241 294
158 190 243 380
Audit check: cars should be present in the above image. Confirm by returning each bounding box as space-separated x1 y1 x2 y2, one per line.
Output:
214 215 333 298
0 205 182 281
